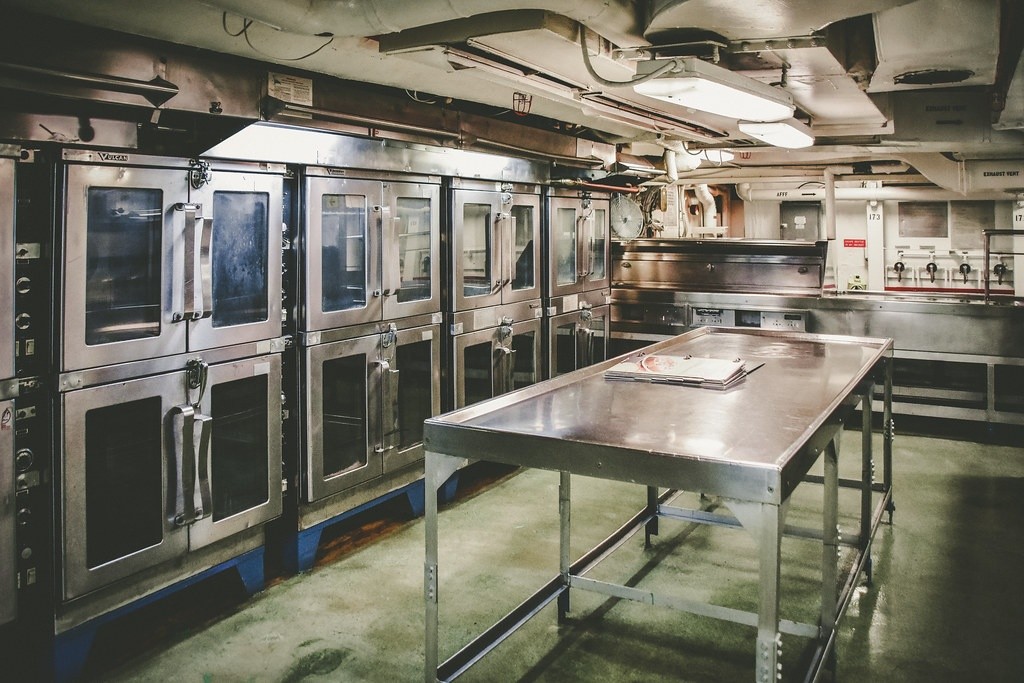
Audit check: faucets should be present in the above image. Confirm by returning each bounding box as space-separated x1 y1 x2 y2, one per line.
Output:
926 262 938 284
959 263 971 285
894 262 904 282
993 263 1006 285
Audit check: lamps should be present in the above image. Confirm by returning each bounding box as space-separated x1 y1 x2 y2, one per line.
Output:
633 60 796 124
736 119 816 149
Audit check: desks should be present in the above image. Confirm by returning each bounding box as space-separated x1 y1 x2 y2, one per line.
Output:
423 325 892 683
